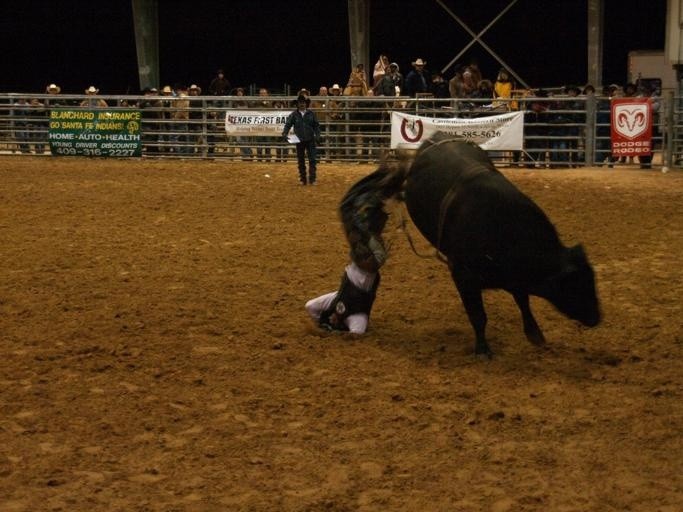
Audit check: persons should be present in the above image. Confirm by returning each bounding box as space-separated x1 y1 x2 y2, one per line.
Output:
305 147 409 341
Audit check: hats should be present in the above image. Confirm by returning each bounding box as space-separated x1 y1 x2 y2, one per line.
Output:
161 86 173 94
455 64 464 73
641 86 656 93
85 86 99 94
187 84 201 94
47 84 60 94
294 88 310 108
412 59 427 66
564 85 581 95
624 83 637 94
329 84 342 94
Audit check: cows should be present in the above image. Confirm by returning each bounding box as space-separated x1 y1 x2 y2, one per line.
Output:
402 129 601 363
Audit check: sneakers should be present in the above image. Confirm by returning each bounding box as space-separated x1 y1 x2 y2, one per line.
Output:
299 180 319 185
